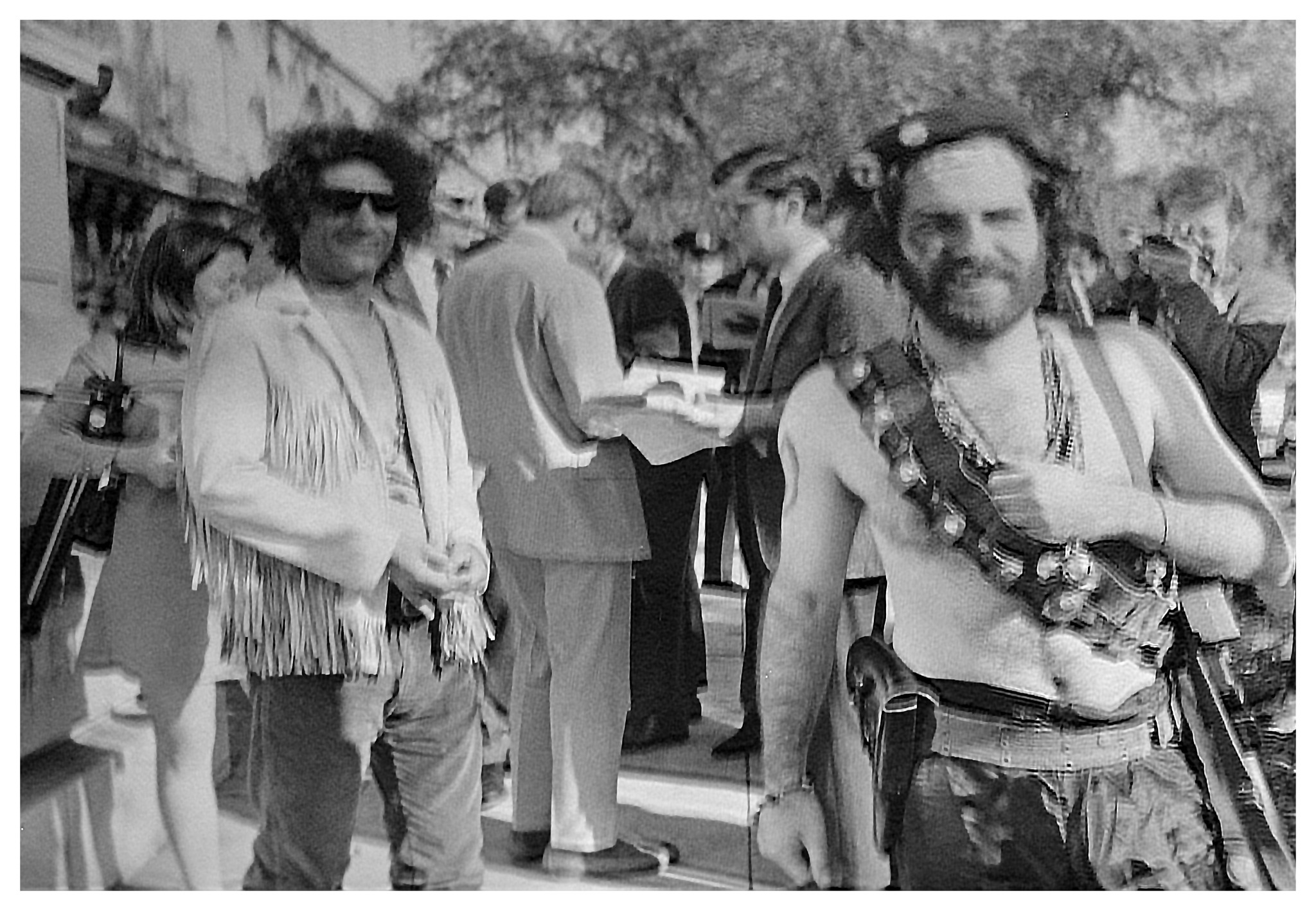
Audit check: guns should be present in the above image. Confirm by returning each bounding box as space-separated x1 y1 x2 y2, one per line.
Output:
845 636 939 856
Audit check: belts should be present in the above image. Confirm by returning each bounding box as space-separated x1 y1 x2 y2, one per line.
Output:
384 586 442 626
927 700 1179 775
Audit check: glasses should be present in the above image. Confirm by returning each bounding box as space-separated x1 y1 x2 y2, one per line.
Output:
310 182 405 217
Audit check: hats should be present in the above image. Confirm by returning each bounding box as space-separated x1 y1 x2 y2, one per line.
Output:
883 96 1079 184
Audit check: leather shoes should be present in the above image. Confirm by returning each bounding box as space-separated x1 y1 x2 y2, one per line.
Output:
620 709 689 751
679 697 703 719
709 715 764 759
543 833 681 877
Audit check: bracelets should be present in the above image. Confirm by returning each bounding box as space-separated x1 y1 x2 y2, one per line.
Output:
754 773 816 830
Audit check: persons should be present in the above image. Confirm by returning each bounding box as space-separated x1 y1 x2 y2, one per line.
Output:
752 112 1295 893
1092 163 1291 480
176 126 498 892
658 147 915 586
16 218 261 892
458 180 1112 767
432 168 678 881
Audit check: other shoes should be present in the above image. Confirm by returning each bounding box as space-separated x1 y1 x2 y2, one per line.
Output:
700 579 746 598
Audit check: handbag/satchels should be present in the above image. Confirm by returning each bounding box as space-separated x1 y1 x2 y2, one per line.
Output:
66 325 132 558
